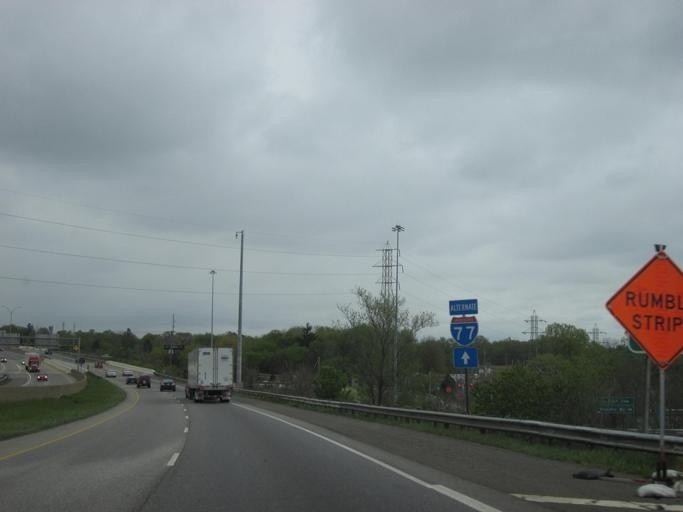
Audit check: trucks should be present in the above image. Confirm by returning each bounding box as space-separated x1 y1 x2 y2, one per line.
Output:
24 352 41 372
184 346 234 403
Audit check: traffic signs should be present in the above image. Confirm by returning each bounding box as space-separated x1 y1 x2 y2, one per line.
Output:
449 315 479 348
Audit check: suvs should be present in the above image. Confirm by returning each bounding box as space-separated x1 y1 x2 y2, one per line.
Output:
136 375 151 388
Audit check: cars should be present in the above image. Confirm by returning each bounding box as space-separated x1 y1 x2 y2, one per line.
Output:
159 378 176 392
104 368 134 377
0 356 8 363
75 357 86 364
126 376 137 385
36 372 48 381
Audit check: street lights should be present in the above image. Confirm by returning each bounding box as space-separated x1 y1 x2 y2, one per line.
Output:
0 304 23 336
